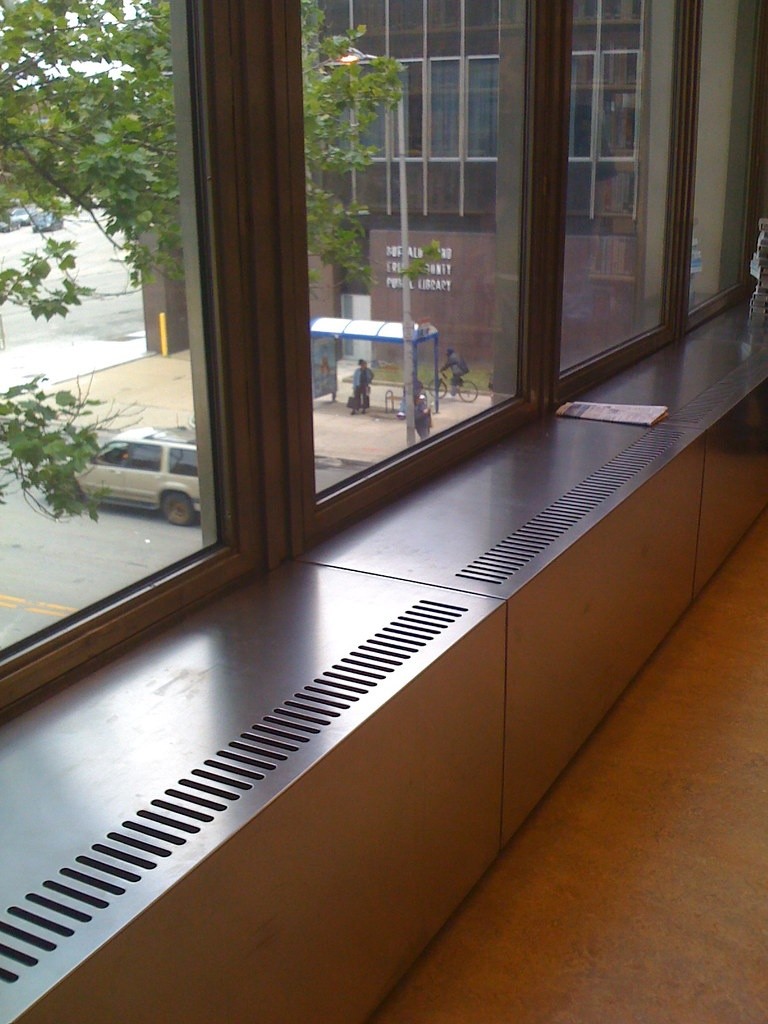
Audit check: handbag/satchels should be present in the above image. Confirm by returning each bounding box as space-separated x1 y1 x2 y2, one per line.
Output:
346 397 360 411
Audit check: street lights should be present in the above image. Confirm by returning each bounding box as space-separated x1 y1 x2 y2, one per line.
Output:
335 45 415 449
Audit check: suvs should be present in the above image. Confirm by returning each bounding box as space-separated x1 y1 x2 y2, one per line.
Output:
50 425 200 527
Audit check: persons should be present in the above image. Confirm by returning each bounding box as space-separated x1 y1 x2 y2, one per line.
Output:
397 370 434 441
351 359 374 415
441 347 466 396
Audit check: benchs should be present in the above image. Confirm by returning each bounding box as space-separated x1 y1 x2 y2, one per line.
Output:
384 390 427 412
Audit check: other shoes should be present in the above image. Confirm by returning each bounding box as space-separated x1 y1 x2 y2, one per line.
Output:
362 410 366 414
350 411 356 415
450 390 456 396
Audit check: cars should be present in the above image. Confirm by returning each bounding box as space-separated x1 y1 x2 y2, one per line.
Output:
0 203 65 233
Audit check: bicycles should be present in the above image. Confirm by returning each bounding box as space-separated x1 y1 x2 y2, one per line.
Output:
427 370 479 403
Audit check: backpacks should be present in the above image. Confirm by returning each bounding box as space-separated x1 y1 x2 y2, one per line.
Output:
457 354 468 373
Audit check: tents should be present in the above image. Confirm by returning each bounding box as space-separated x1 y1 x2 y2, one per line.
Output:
309 317 439 414
